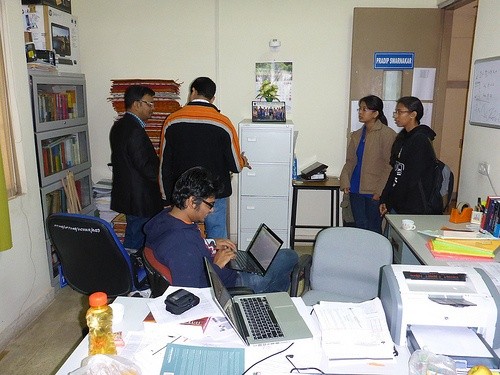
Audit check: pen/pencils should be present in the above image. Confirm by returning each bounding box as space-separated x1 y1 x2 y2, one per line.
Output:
475 198 485 212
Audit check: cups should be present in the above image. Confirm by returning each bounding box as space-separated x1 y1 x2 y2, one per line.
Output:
402 219 415 231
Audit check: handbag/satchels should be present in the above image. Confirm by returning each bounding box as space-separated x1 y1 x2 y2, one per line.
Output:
164 289 200 315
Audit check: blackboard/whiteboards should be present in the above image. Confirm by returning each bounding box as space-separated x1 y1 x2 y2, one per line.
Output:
469 56 500 129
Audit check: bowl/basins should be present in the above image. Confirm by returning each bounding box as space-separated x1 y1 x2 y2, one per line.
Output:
108 303 125 323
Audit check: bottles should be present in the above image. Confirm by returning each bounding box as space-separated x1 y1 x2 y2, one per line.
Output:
85 292 117 357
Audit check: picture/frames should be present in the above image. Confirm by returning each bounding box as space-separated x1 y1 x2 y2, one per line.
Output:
252 101 286 122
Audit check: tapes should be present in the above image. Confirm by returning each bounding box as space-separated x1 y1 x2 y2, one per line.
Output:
457 202 470 214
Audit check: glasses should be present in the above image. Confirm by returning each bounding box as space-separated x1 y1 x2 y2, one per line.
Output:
357 109 377 113
141 101 155 106
202 200 215 209
393 111 411 114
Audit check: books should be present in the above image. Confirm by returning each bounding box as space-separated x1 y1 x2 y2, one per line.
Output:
37 90 120 262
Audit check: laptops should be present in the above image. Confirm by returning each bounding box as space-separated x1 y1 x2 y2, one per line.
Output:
230 224 283 277
204 256 313 347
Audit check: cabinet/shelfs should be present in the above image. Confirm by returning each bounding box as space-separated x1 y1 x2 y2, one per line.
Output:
30 71 98 287
238 119 294 252
384 214 500 268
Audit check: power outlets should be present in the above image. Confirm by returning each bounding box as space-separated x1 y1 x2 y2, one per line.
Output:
478 161 489 175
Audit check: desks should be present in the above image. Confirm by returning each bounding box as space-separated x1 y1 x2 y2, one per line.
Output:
291 176 342 250
55 295 407 375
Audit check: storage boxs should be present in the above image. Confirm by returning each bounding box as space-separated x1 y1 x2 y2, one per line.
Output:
21 0 79 69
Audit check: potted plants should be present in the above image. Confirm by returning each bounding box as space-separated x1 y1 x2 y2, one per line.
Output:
257 80 281 102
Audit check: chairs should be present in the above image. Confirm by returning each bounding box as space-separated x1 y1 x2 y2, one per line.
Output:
290 226 393 307
142 246 256 299
46 213 150 298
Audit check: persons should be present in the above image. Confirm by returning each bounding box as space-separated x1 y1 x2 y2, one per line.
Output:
340 94 436 236
110 85 163 249
253 106 284 120
144 166 306 294
158 77 251 239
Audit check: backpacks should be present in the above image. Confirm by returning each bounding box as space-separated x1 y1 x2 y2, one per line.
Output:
407 133 454 215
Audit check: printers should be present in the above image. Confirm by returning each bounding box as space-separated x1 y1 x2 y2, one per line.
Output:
379 265 500 370
